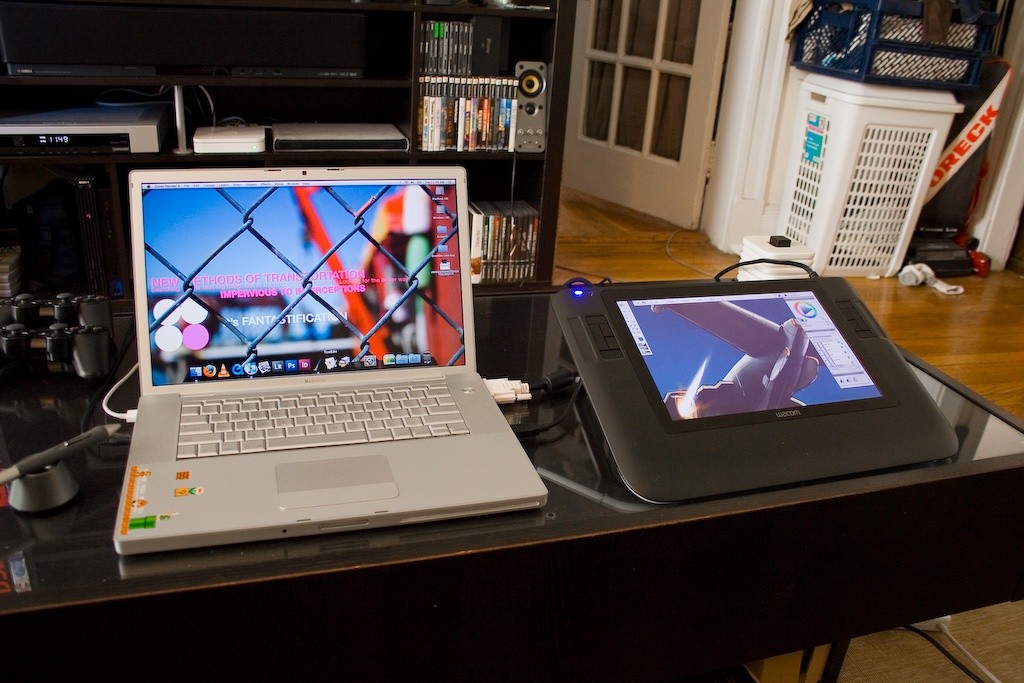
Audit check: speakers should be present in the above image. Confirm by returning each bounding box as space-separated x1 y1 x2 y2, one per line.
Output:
516 61 546 153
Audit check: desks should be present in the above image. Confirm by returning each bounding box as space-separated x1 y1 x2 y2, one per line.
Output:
1 282 1024 683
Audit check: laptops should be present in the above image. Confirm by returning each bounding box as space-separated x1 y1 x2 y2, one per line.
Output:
114 164 549 554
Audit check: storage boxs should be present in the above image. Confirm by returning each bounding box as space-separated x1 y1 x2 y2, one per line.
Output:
767 68 964 277
799 1 1001 90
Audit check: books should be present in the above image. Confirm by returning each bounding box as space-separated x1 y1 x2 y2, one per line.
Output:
468 199 539 285
418 76 519 153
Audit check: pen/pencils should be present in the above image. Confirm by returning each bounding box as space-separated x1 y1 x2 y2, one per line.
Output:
0 423 122 485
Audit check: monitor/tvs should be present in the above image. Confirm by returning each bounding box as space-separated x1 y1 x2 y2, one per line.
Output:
551 276 959 505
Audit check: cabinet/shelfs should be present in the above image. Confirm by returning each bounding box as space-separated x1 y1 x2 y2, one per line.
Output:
0 0 577 284
